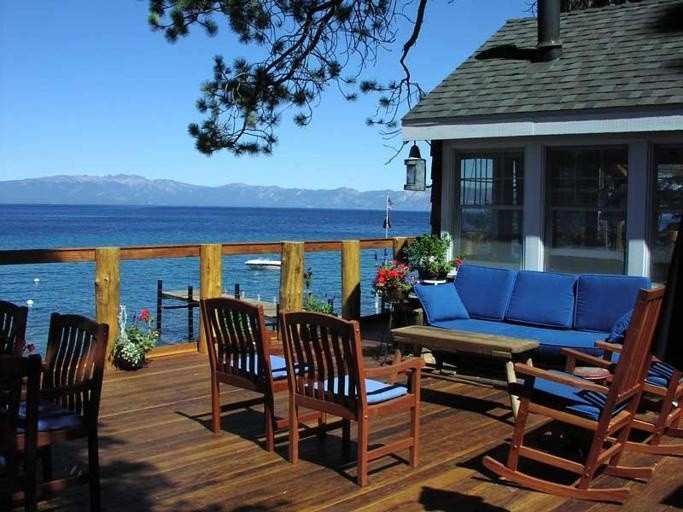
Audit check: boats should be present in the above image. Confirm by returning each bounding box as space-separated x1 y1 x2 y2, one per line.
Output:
243 257 281 270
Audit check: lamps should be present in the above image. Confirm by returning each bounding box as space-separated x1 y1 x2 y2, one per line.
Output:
402 140 426 193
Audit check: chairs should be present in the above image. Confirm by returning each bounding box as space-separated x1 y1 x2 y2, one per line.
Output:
0 300 108 511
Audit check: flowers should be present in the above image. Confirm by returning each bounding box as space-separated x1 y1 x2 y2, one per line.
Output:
397 233 463 274
106 303 160 367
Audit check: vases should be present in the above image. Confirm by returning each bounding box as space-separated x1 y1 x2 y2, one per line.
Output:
117 356 144 370
